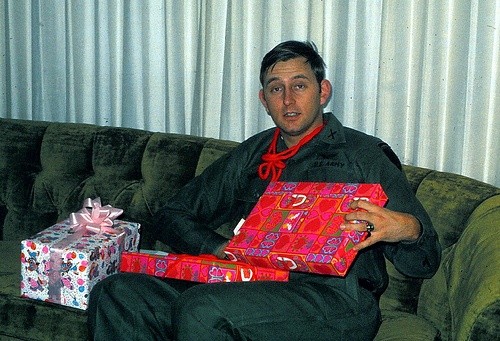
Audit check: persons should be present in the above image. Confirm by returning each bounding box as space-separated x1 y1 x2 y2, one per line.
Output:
87 39 442 341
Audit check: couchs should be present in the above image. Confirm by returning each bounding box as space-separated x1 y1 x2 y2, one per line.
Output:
0 117 500 341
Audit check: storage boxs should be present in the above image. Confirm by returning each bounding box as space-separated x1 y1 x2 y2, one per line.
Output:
119 249 290 284
222 179 389 278
18 196 143 310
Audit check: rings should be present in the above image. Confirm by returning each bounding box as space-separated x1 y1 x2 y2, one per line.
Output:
366 223 374 232
